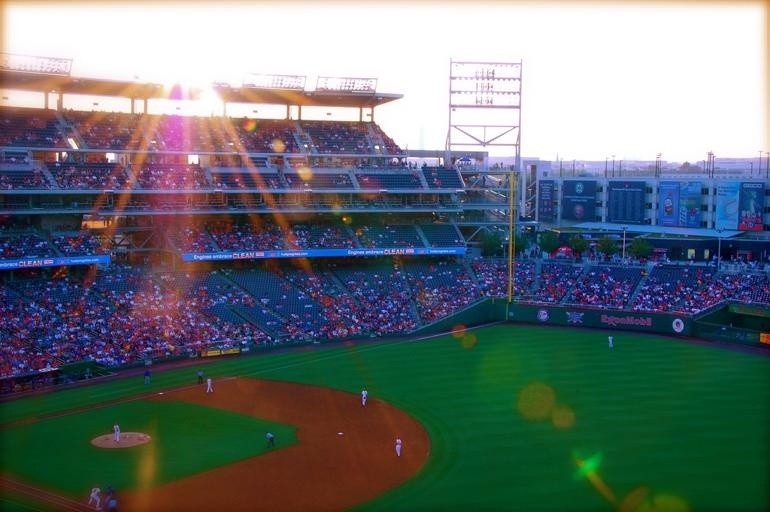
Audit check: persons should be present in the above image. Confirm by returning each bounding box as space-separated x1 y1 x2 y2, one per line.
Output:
396 437 402 457
362 388 369 407
495 162 770 352
1 107 495 392
112 423 120 442
89 485 118 510
266 432 275 447
